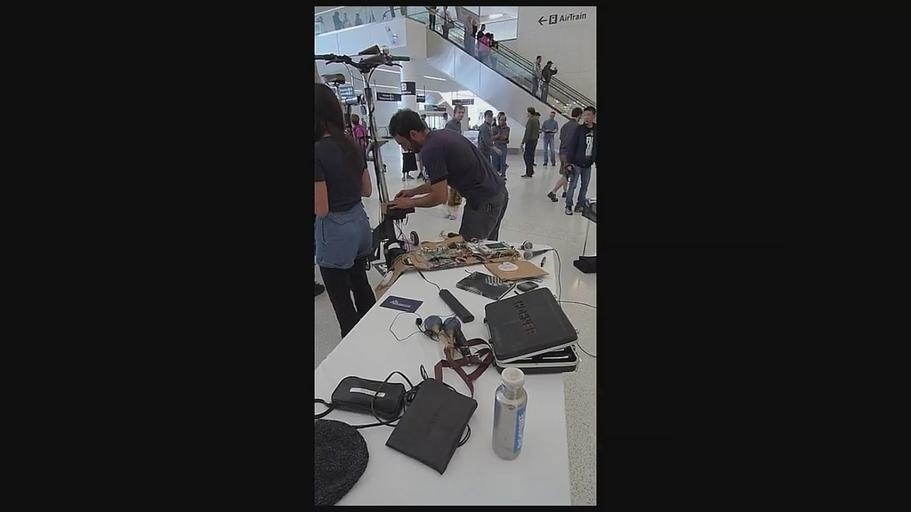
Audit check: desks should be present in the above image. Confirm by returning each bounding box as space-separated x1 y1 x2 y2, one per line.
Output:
315 242 572 505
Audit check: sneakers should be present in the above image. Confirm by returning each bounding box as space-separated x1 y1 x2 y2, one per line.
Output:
565 204 588 215
401 175 415 181
547 192 567 202
543 163 555 167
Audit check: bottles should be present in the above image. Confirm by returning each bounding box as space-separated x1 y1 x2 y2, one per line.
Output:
490 365 528 461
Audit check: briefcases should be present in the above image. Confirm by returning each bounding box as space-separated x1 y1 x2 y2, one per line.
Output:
483 285 581 377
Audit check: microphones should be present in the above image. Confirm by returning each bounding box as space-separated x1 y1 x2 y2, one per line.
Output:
523 249 553 260
522 239 533 249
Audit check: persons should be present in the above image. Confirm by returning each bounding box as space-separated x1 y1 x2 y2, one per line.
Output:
520 107 540 179
540 61 559 104
385 106 510 241
563 105 597 216
351 114 369 156
533 111 544 167
542 110 559 167
546 107 584 203
333 5 500 72
395 105 511 221
531 55 544 97
314 80 378 341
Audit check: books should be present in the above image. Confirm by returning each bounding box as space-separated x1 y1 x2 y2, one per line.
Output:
456 271 516 302
386 379 478 473
